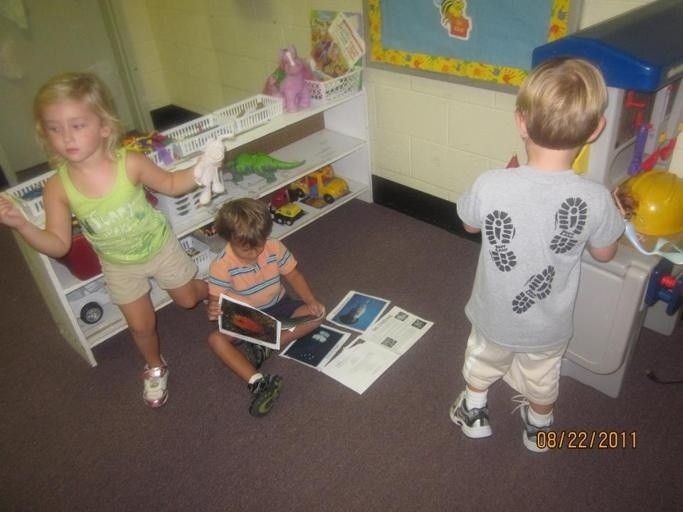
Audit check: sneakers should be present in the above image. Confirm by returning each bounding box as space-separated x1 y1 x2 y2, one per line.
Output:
142 353 170 410
235 341 272 372
247 371 283 418
449 390 493 439
520 397 553 453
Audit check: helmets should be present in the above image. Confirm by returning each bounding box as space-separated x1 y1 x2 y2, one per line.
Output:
621 170 683 235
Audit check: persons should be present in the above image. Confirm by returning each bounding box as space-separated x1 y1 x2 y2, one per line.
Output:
447 56 624 453
0 72 223 409
204 200 324 416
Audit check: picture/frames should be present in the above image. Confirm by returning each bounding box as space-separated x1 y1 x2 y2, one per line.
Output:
362 0 583 94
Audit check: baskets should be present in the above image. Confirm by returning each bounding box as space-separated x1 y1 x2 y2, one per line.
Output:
211 92 284 136
150 186 213 234
5 169 63 217
303 66 363 105
179 236 210 271
148 142 174 171
158 112 235 160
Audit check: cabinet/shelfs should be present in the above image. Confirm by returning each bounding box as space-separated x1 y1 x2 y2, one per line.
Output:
0 84 373 367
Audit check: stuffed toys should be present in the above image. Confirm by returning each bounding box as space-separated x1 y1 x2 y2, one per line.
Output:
262 46 310 115
